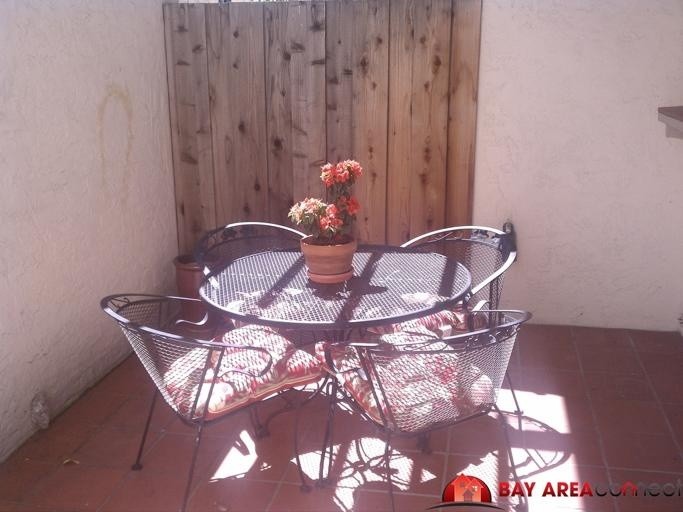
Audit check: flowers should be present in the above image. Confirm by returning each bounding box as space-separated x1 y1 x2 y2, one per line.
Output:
287 160 364 244
